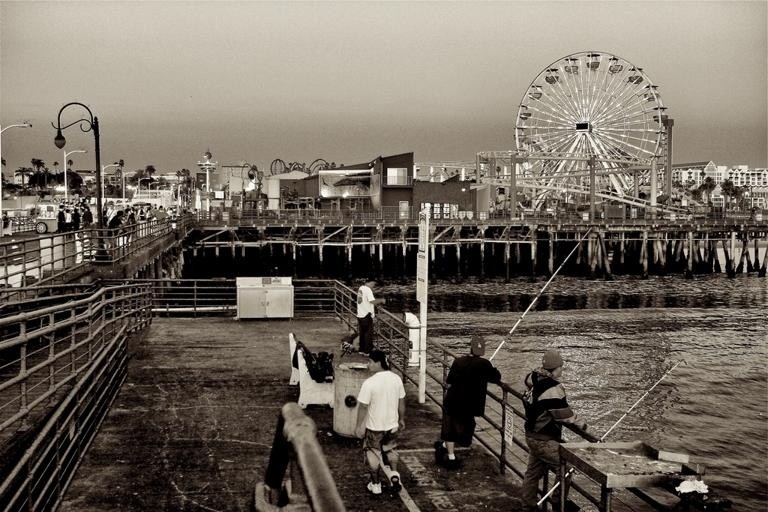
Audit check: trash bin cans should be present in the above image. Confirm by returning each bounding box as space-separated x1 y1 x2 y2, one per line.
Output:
333 355 375 438
404 313 420 366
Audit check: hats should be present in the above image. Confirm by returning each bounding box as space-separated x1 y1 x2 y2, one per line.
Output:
471 335 485 356
369 350 388 371
542 349 563 370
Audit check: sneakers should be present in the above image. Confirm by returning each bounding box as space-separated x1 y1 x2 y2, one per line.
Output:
367 480 382 495
387 472 402 492
435 441 457 466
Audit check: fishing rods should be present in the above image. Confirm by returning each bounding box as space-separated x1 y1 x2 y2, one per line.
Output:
534 357 684 508
489 223 594 363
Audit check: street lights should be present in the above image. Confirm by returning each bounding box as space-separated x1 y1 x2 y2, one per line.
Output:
102 163 120 208
62 149 87 206
136 177 166 202
1 122 28 211
53 100 113 263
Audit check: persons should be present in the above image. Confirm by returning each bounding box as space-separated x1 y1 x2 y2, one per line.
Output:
354 348 408 495
356 279 386 358
521 350 597 512
56 204 198 257
2 210 13 237
433 332 501 470
